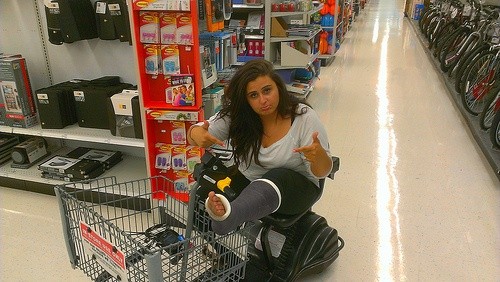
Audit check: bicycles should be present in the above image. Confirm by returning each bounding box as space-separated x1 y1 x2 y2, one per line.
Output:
418 0 500 149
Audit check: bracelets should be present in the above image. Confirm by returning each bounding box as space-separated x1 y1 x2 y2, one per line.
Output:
189 125 200 141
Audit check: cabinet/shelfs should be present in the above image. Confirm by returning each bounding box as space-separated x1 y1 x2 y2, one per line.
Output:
0 0 366 230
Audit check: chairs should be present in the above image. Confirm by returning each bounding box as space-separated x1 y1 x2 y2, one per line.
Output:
258 156 340 229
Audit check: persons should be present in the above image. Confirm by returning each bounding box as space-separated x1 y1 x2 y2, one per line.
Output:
174 83 195 106
186 60 333 235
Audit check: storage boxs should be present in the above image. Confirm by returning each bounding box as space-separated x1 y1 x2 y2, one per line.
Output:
0 53 39 129
281 42 311 67
